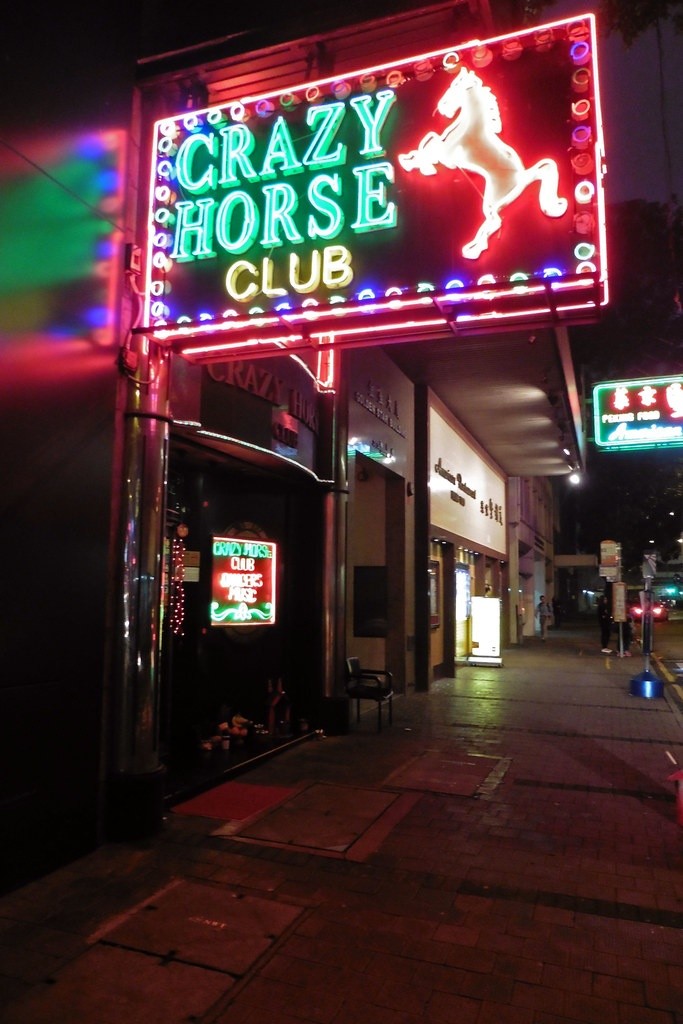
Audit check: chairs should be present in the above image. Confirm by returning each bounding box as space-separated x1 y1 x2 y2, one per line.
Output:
346 656 393 731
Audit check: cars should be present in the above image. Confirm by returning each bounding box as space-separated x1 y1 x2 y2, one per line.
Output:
629 597 668 621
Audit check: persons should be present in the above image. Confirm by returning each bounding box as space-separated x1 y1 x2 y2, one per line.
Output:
535 595 553 639
617 618 632 653
597 595 612 653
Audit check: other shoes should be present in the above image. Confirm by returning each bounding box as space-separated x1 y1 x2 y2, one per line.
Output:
600 648 613 652
616 651 632 656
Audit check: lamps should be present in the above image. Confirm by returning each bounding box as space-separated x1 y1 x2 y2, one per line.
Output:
548 395 576 472
